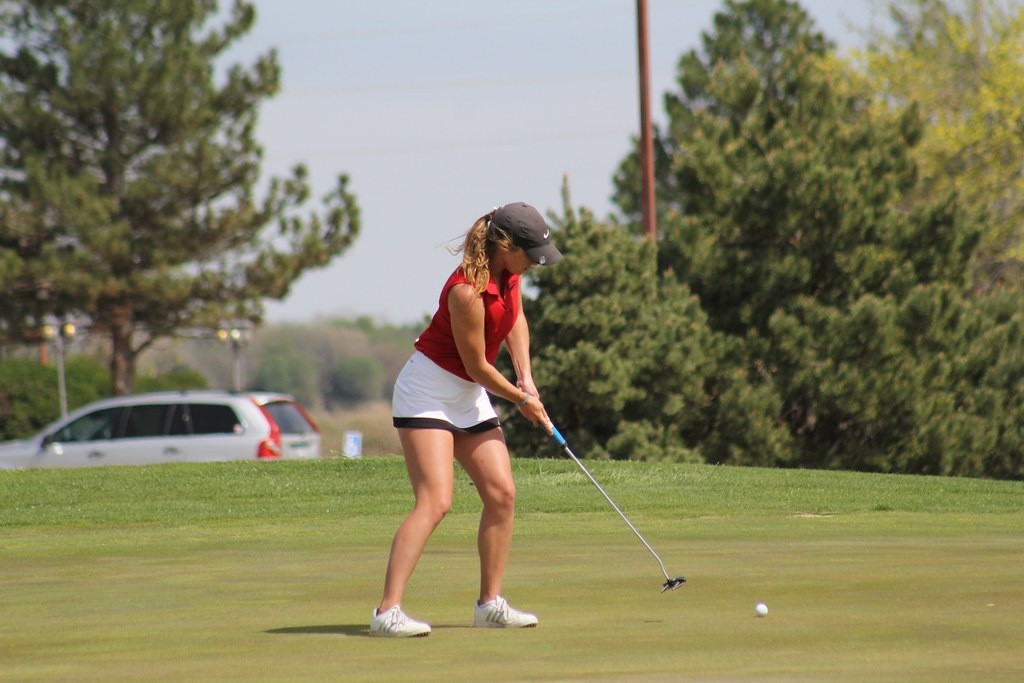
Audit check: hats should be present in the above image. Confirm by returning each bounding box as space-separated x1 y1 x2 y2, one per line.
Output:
489 201 565 266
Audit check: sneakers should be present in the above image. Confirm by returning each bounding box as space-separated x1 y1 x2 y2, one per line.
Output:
369 606 430 638
475 594 539 628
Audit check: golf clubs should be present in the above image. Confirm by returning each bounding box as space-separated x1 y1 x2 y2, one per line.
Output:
517 383 687 595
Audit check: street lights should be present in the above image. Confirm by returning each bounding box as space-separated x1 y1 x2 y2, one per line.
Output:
42 319 76 439
219 328 242 390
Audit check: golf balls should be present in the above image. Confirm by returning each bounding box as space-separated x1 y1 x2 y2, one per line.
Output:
755 604 769 616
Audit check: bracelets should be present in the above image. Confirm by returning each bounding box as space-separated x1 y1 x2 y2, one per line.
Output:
516 392 529 408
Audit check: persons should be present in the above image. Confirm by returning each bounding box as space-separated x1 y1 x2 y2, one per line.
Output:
367 202 565 637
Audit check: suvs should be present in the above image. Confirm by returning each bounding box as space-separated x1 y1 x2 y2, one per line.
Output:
0 388 322 468
